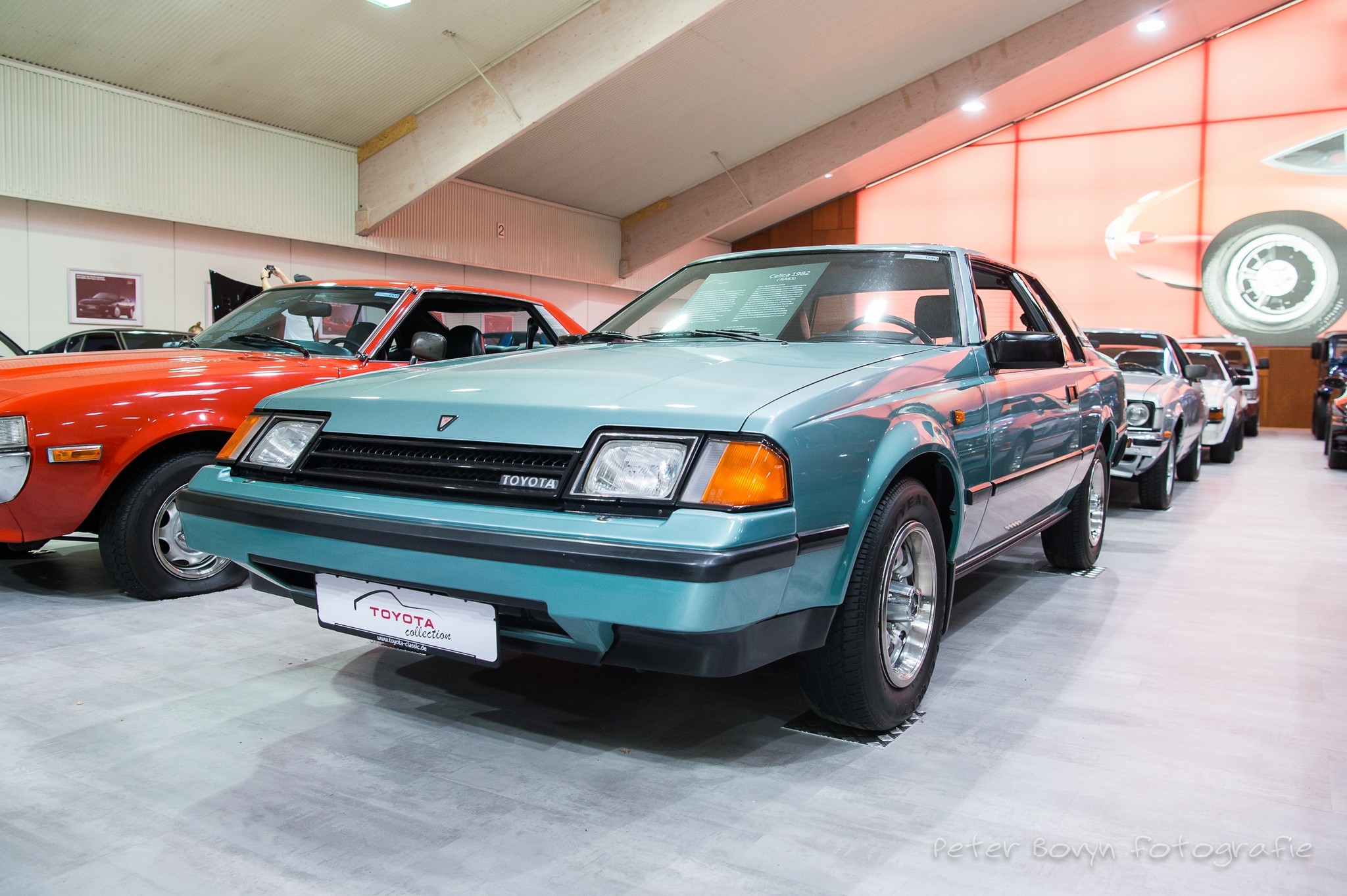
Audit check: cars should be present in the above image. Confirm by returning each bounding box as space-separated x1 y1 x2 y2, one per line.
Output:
1308 330 1347 470
1172 333 1271 436
1180 349 1251 464
1 276 580 602
173 239 1130 736
1080 328 1209 511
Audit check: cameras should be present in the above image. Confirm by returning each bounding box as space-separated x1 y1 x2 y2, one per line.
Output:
266 265 274 273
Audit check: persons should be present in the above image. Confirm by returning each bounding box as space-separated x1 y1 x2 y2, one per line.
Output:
187 322 204 335
260 263 323 342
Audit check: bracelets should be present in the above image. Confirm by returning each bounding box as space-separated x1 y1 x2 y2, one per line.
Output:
261 276 267 280
